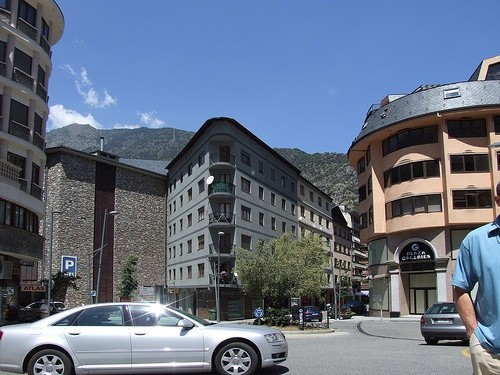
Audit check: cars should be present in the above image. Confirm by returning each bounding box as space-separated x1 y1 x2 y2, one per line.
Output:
0 302 289 375
293 306 323 322
7 300 65 322
338 300 367 316
420 301 476 346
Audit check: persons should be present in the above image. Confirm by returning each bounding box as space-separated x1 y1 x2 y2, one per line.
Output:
39 299 53 319
450 181 500 375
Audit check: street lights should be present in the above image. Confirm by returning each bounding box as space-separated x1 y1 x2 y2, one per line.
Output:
217 231 225 322
48 207 64 302
95 209 121 304
